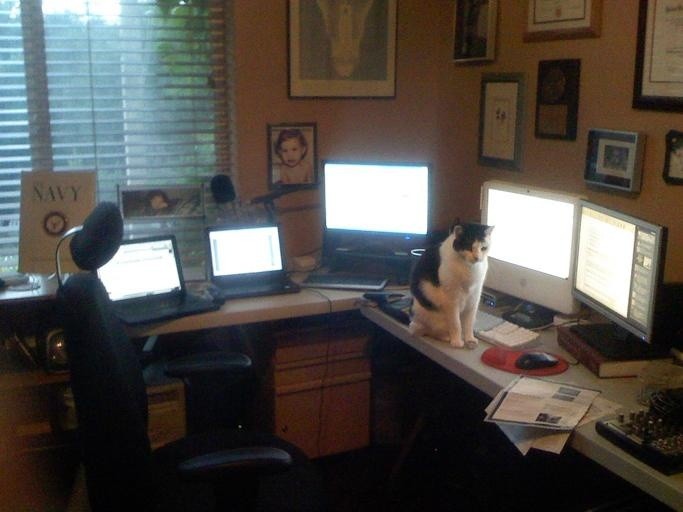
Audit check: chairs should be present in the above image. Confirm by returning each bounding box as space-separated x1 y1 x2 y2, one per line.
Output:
50 199 308 508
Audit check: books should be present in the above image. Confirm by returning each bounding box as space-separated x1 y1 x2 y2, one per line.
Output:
558 326 676 379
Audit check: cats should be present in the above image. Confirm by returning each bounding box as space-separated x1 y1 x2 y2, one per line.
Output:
364 222 495 349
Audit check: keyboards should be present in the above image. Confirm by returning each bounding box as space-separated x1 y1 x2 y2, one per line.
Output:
474 310 540 352
300 272 390 291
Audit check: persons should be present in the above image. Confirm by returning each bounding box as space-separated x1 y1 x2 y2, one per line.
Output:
274 129 313 184
137 190 169 216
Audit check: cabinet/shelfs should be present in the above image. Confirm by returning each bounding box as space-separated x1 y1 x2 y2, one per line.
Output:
249 331 371 460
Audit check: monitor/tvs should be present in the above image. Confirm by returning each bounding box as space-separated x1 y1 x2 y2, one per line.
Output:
571 199 668 358
322 160 432 277
481 180 587 329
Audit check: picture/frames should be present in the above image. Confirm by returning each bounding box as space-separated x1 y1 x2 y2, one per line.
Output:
630 2 683 113
581 126 647 195
533 57 581 141
284 0 399 101
449 0 499 69
263 120 322 189
520 2 602 43
476 69 526 172
663 129 683 185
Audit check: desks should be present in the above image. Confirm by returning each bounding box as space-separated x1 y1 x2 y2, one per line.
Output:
360 288 680 509
1 267 411 512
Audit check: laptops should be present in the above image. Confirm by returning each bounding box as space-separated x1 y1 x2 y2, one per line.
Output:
95 234 219 324
206 223 299 299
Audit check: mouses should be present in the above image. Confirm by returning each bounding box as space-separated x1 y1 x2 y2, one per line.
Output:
515 352 557 368
